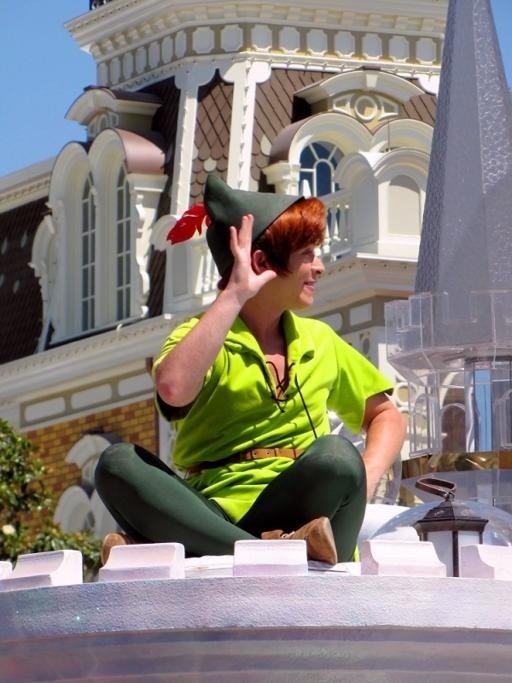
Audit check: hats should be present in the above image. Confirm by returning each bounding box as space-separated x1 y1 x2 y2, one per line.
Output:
204 175 304 277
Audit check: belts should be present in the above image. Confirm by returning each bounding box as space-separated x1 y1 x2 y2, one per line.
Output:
189 445 307 472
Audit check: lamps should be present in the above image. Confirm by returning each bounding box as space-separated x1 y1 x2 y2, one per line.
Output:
414 491 489 577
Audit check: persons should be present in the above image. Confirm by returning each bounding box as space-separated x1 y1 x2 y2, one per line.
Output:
90 170 411 570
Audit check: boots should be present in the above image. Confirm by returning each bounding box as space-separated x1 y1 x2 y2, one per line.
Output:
101 532 138 565
261 516 338 564
